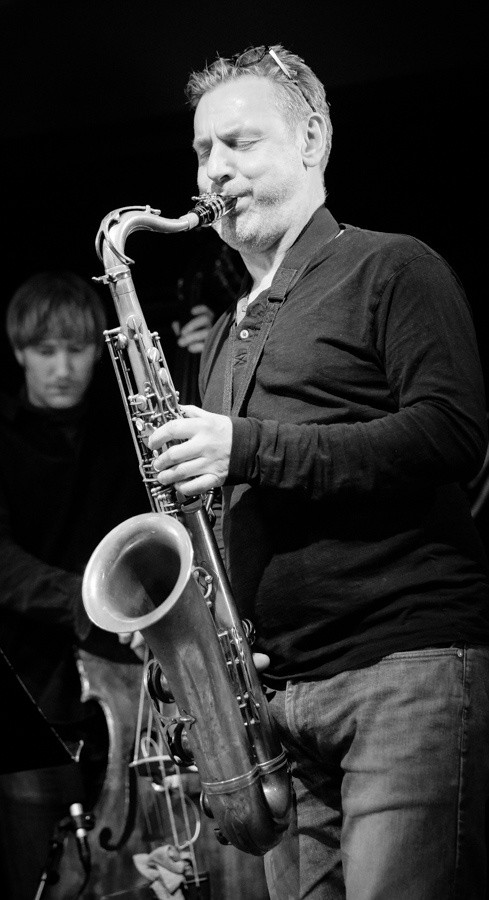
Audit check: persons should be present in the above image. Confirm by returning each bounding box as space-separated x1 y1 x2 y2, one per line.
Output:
149 46 489 900
0 278 215 900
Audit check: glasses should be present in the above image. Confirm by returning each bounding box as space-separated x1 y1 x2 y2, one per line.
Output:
205 46 317 114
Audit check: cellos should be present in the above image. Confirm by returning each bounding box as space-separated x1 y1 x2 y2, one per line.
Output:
34 231 271 900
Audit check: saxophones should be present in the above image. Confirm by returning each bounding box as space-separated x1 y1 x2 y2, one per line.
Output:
81 189 300 857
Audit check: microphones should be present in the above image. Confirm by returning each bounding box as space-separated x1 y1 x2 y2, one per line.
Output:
68 801 92 873
